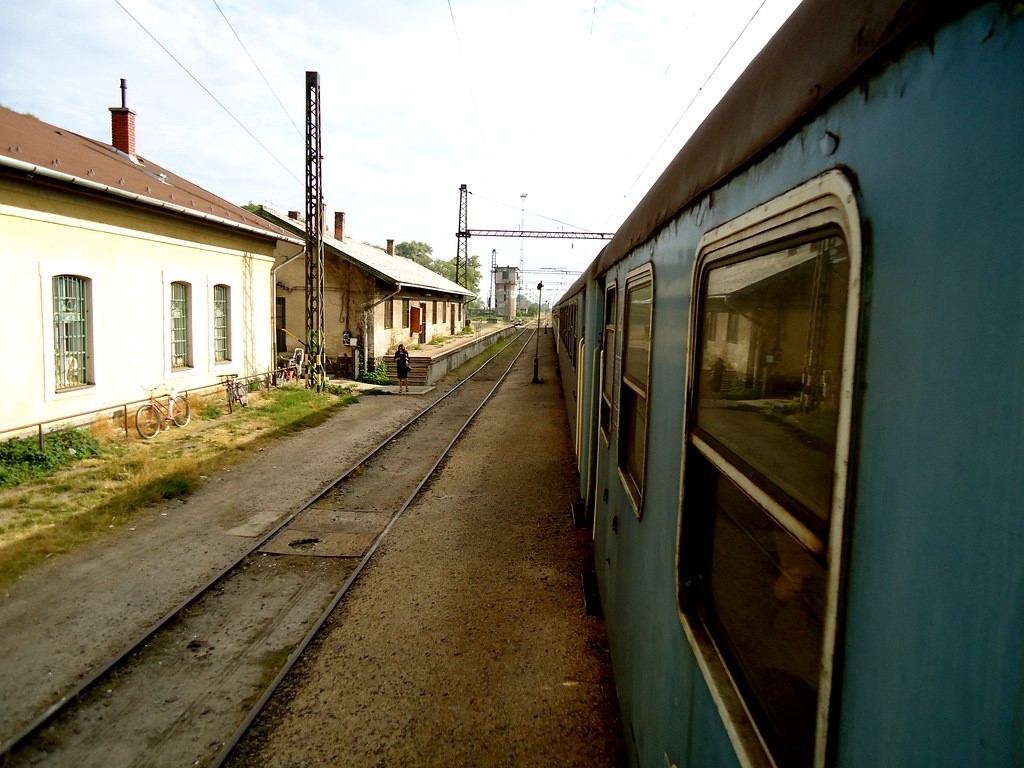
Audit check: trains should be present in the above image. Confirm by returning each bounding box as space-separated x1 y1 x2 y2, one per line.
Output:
547 0 1024 768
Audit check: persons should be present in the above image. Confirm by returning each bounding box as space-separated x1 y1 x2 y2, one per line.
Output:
710 358 727 392
394 344 409 393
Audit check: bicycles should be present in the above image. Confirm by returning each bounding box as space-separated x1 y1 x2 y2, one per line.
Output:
216 373 249 414
277 356 300 388
135 383 191 439
